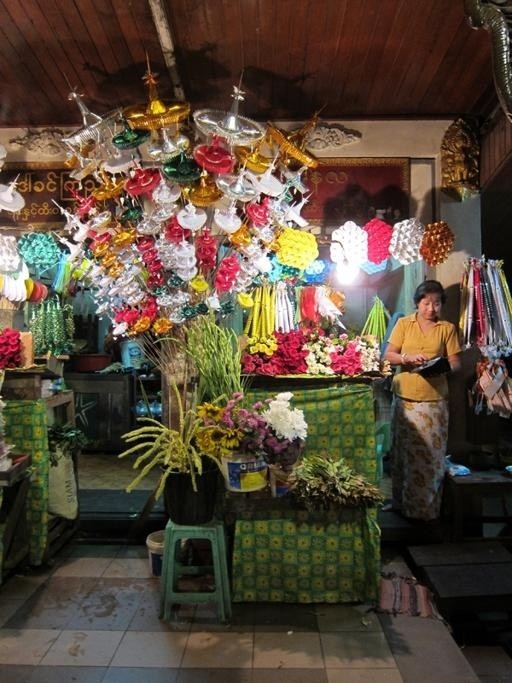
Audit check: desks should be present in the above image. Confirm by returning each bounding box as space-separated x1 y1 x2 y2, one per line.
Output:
227 491 382 604
243 375 382 489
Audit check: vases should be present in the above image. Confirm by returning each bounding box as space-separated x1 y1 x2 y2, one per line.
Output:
71 354 111 373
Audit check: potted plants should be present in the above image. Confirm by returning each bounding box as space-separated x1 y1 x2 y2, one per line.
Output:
117 379 226 526
104 325 146 368
286 453 386 514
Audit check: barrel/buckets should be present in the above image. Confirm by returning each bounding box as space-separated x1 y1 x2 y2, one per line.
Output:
146 529 189 579
119 339 146 369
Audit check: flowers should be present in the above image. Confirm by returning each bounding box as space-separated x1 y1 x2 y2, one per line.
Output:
203 391 308 465
0 328 21 368
241 327 383 378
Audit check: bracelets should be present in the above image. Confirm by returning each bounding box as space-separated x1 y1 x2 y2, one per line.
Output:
401 353 409 365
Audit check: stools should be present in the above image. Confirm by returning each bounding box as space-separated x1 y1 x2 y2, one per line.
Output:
160 518 232 626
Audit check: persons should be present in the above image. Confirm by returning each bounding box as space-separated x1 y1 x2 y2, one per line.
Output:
382 280 463 521
